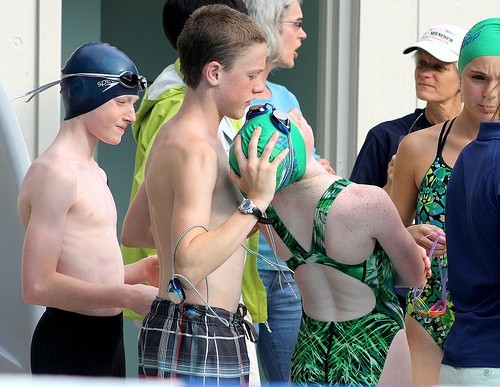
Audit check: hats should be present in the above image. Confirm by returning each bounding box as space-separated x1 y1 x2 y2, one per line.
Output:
61 40 140 121
228 107 307 200
403 24 469 62
459 17 500 77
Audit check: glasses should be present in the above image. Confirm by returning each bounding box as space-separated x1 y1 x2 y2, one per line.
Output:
165 224 296 327
277 18 303 28
412 228 448 315
245 102 295 190
11 70 149 104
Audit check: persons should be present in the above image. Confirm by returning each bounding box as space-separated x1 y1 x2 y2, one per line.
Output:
439 122 500 387
121 0 335 387
121 4 289 386
17 42 159 378
348 32 462 189
388 19 500 387
229 103 432 387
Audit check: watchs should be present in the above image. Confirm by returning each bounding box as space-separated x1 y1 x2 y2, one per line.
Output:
236 197 278 224
